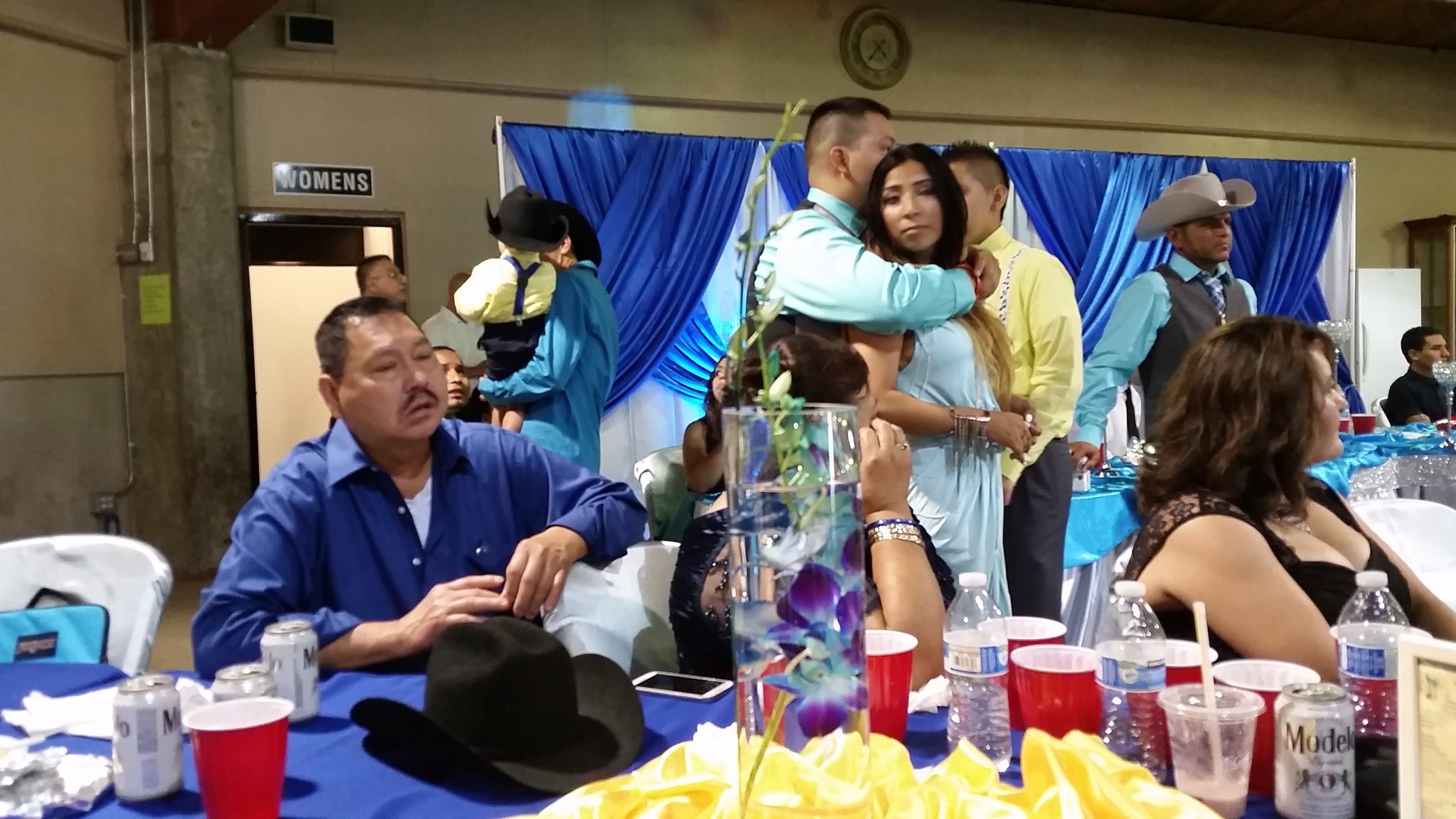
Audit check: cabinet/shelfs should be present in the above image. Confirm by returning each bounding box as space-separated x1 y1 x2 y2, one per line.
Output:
1402 213 1454 361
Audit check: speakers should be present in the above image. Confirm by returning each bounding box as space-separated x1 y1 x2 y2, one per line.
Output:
286 11 340 52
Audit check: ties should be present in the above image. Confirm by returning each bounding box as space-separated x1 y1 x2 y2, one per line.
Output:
1198 274 1226 317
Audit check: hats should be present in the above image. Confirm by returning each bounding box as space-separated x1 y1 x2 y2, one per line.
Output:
347 615 645 794
482 186 569 252
1136 172 1256 244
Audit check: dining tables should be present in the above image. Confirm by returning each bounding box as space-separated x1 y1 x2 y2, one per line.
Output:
0 661 1276 819
1054 425 1456 649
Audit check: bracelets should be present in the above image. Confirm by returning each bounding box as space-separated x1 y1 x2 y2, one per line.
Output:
866 517 926 548
958 262 981 298
946 403 991 464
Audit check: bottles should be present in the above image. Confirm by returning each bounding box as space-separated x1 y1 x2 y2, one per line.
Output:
941 574 1016 772
1092 578 1171 788
1333 569 1416 819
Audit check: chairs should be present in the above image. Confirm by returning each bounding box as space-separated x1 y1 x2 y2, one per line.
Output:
543 540 690 688
633 444 686 495
0 534 171 681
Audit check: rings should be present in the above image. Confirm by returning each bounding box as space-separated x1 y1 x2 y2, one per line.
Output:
1067 448 1073 454
1022 430 1026 439
896 441 909 450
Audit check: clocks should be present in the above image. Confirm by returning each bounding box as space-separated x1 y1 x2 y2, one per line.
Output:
840 3 912 91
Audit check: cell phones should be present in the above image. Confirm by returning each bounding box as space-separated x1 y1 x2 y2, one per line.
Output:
631 670 734 703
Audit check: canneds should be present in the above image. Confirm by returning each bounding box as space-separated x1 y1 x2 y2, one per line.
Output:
210 661 279 702
1272 682 1356 819
112 673 182 802
260 620 320 722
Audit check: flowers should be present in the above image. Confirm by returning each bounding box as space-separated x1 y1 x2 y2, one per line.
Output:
721 98 865 818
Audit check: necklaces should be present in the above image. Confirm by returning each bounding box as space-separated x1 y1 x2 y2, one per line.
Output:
1265 492 1313 535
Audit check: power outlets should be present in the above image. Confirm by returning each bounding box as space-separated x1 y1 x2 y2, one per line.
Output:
140 239 153 262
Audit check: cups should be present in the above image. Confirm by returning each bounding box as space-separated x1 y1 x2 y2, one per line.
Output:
1331 619 1434 740
1010 641 1104 746
1156 682 1267 819
1211 656 1322 799
850 627 920 747
1352 412 1380 437
1337 416 1351 435
1100 635 1221 776
977 613 1069 732
181 687 296 819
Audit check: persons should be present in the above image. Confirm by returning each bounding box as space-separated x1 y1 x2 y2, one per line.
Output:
1383 327 1456 425
192 296 652 688
680 355 733 514
742 89 1002 353
668 331 960 691
939 141 1088 623
1109 313 1456 691
348 185 622 478
1065 172 1260 481
844 136 1042 635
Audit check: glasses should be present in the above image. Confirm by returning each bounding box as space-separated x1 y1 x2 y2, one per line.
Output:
369 273 410 284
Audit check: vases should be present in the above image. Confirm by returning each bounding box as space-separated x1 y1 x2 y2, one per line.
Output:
718 402 865 819
